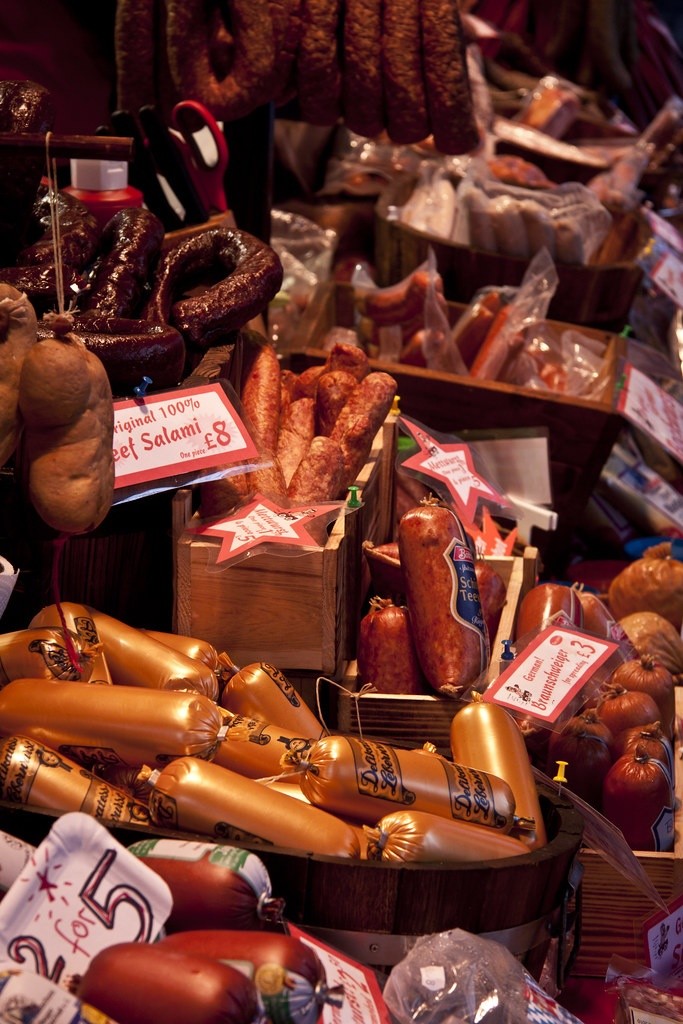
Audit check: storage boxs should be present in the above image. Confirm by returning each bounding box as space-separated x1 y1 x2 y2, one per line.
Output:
1 72 682 1024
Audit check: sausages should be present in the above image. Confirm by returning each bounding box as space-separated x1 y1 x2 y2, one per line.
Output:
0 0 683 1020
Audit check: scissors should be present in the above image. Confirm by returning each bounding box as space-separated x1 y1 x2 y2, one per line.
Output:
143 99 228 214
